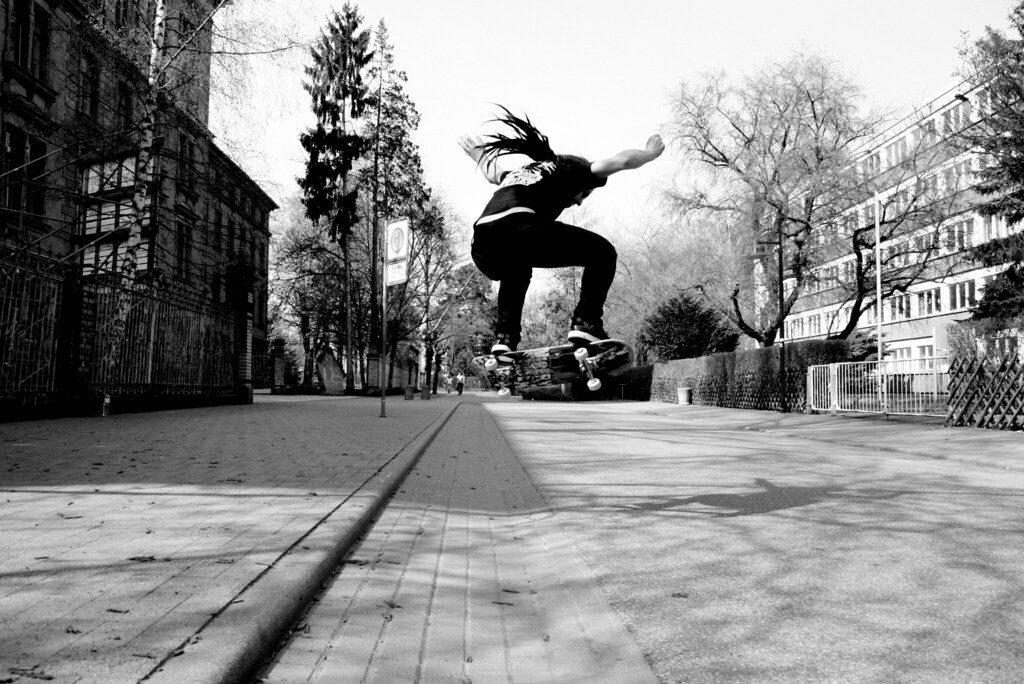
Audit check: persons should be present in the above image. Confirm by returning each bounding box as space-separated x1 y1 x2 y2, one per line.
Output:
446 375 454 394
292 368 301 395
456 102 665 356
456 372 465 395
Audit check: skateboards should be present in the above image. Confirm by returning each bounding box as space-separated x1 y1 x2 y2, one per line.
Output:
469 338 634 401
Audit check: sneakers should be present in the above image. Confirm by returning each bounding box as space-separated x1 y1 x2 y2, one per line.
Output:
491 331 521 354
568 315 610 344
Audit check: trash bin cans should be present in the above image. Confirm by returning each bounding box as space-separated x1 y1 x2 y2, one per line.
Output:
677 387 692 404
